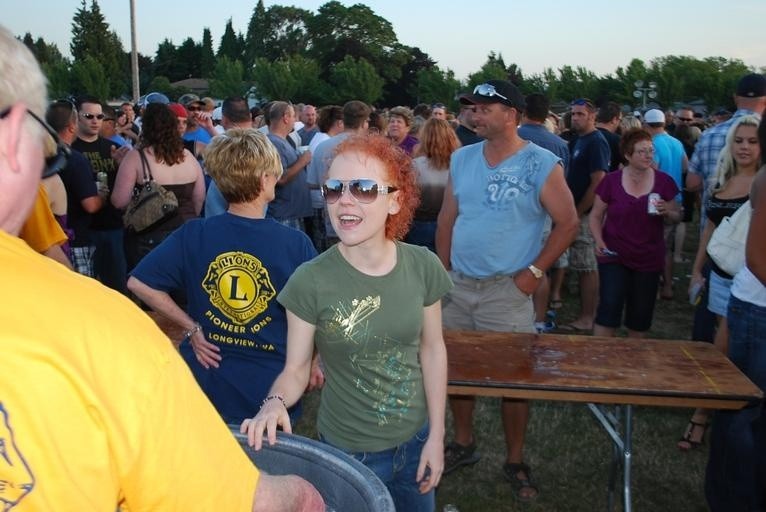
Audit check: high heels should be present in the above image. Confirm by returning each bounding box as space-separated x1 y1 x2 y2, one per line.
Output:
675 416 711 453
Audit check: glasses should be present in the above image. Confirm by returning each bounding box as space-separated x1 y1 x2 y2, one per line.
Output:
320 177 401 207
1 104 72 179
676 115 693 122
570 98 592 107
631 148 657 157
79 109 107 124
471 83 508 102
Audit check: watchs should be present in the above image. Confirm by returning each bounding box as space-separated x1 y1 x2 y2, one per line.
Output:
529 264 544 280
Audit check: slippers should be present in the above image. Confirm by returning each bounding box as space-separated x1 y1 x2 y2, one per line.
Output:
550 296 565 310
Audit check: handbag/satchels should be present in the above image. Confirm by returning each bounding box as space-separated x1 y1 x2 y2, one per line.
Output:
122 150 179 238
705 199 751 281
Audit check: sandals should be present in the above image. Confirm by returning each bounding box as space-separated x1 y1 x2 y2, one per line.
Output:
504 460 541 503
445 440 480 471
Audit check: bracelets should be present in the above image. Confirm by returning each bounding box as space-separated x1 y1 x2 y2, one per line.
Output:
183 322 202 338
259 395 287 410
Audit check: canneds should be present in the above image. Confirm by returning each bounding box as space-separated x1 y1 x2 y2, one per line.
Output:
97 172 107 193
648 191 662 216
299 146 310 157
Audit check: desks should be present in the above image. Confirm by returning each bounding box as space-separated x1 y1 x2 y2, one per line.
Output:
420 326 760 512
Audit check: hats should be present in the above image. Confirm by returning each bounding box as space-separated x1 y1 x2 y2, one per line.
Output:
138 92 169 110
174 105 190 124
632 111 641 116
643 109 667 125
734 74 765 100
177 93 205 106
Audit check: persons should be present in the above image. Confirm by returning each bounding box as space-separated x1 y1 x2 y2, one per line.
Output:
369 106 390 131
212 107 223 126
41 129 74 258
645 109 688 300
71 95 130 298
595 102 629 173
201 97 215 113
677 114 761 453
17 181 74 273
309 101 371 249
456 107 465 126
250 99 267 127
45 98 110 278
665 107 711 142
296 105 319 146
588 127 681 339
566 98 612 331
436 79 579 503
383 106 420 158
431 103 447 119
400 118 462 254
109 102 206 312
168 104 221 161
178 94 211 189
704 108 766 512
686 73 766 343
305 106 344 254
127 126 323 434
110 92 169 149
0 23 327 512
257 97 302 147
517 93 570 178
559 112 579 153
544 113 559 134
264 101 306 173
672 123 694 264
266 102 311 231
455 95 486 146
205 97 268 219
240 131 454 512
293 103 304 130
